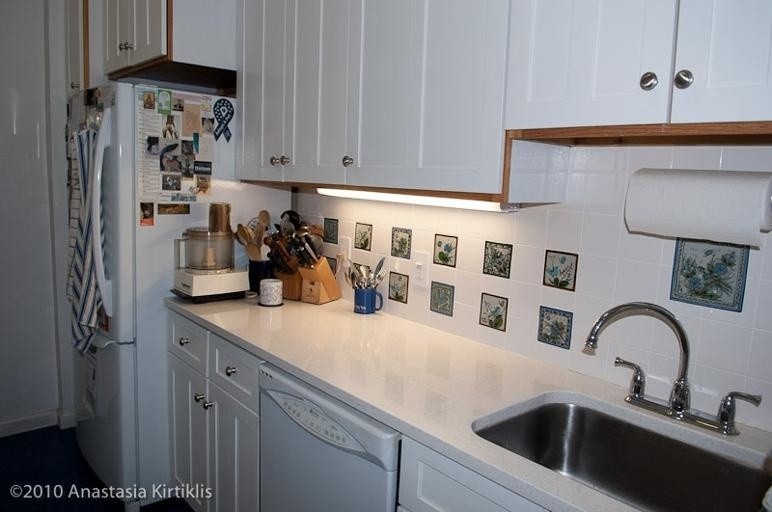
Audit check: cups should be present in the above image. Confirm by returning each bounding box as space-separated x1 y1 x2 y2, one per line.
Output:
352 287 383 316
258 278 285 306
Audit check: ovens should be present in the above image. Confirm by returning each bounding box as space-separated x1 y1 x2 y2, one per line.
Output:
255 357 403 512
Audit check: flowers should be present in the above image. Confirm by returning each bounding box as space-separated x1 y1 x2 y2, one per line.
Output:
678 247 738 302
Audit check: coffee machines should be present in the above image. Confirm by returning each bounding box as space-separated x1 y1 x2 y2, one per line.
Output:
168 197 251 305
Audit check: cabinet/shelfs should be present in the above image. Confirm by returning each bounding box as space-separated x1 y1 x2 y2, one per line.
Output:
344 1 572 209
104 1 235 93
235 1 348 187
506 1 771 146
397 436 553 512
64 0 106 99
163 298 260 512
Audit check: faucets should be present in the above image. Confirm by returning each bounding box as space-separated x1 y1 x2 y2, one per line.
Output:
582 301 691 419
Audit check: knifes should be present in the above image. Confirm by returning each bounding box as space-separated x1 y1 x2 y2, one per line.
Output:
344 257 388 287
264 231 296 273
292 235 317 267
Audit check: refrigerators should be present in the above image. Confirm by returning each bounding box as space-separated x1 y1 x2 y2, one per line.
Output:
63 81 293 512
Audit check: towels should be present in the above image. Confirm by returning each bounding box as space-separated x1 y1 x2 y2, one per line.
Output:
60 123 108 359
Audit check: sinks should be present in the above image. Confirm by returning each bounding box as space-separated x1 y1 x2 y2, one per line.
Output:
471 389 772 510
